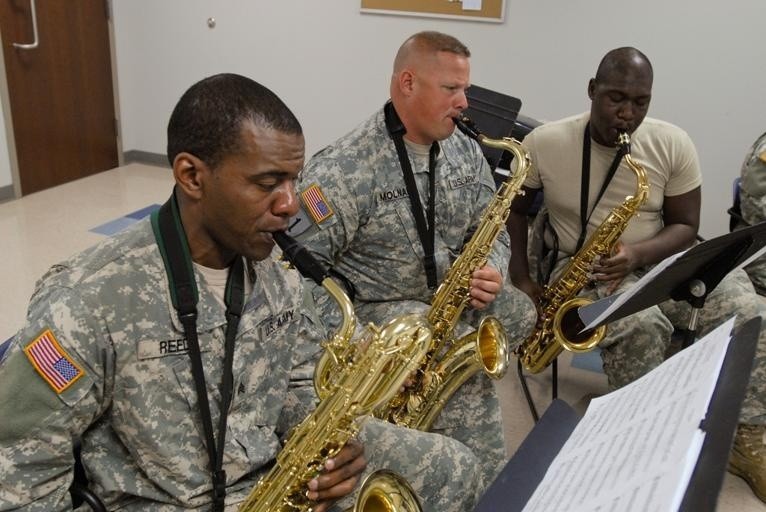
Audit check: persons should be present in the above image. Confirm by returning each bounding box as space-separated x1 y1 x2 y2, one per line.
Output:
507 43 766 507
286 29 540 490
731 132 766 295
0 72 487 511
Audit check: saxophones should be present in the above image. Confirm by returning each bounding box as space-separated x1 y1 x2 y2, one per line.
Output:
373 115 531 432
514 128 650 374
235 229 433 510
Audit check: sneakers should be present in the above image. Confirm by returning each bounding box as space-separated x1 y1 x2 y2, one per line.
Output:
727 418 766 504
